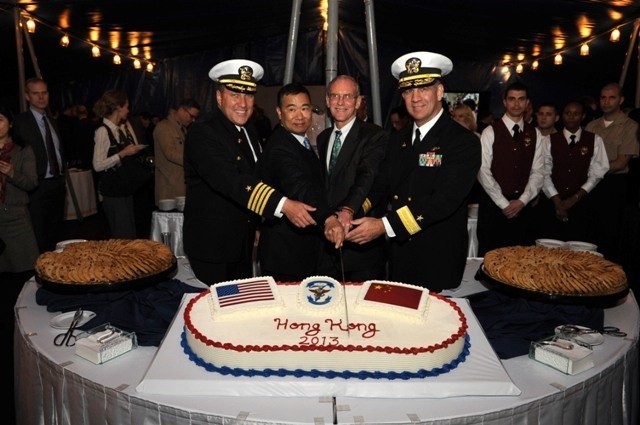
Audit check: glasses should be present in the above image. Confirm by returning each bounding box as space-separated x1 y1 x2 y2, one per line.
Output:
327 92 358 100
184 109 196 118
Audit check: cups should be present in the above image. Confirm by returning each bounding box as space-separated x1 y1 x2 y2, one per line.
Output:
176 196 186 212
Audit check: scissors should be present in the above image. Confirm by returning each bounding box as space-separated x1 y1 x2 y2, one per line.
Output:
581 327 627 337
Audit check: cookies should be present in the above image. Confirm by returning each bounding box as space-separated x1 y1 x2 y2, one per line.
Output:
483 245 627 296
35 239 173 285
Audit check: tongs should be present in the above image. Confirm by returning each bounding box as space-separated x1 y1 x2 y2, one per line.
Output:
561 324 627 340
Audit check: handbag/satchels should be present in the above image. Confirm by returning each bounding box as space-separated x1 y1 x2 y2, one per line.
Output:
64 166 98 221
97 125 152 196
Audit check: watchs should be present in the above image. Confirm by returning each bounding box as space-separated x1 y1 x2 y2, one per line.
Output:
574 193 581 202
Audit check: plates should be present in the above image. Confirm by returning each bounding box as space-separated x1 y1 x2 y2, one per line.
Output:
159 199 176 212
566 241 598 252
49 310 97 329
535 238 565 249
56 238 87 246
554 324 604 346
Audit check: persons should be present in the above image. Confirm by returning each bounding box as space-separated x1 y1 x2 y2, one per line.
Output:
252 83 346 282
541 98 611 242
57 103 73 126
390 106 414 136
314 73 390 283
50 107 60 121
451 102 481 139
13 76 73 254
478 112 496 135
138 111 156 144
534 102 561 135
91 86 145 238
151 115 161 125
181 58 318 286
73 104 94 151
579 95 598 130
463 97 478 121
71 104 79 126
476 82 546 257
345 49 483 295
0 106 42 274
152 97 202 209
583 82 640 263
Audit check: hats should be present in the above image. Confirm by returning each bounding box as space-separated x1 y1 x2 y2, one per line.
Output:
391 51 453 91
208 59 264 94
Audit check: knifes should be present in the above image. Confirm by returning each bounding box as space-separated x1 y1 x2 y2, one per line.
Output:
339 244 349 338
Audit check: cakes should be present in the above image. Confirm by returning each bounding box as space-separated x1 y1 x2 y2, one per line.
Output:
180 275 472 379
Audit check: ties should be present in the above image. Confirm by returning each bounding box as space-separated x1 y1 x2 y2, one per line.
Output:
512 124 520 142
569 134 576 148
303 138 314 153
240 128 256 170
117 128 128 149
328 130 342 177
41 115 60 176
412 128 421 156
